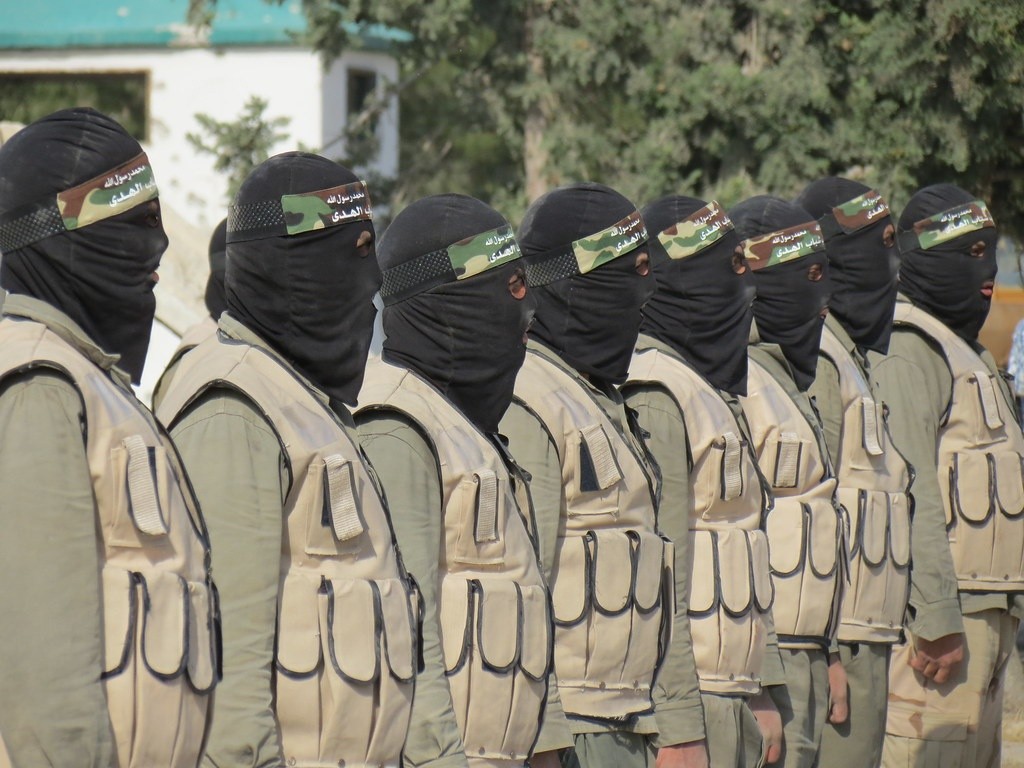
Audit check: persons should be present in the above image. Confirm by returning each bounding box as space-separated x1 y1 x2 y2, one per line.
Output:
0 109 1024 767
